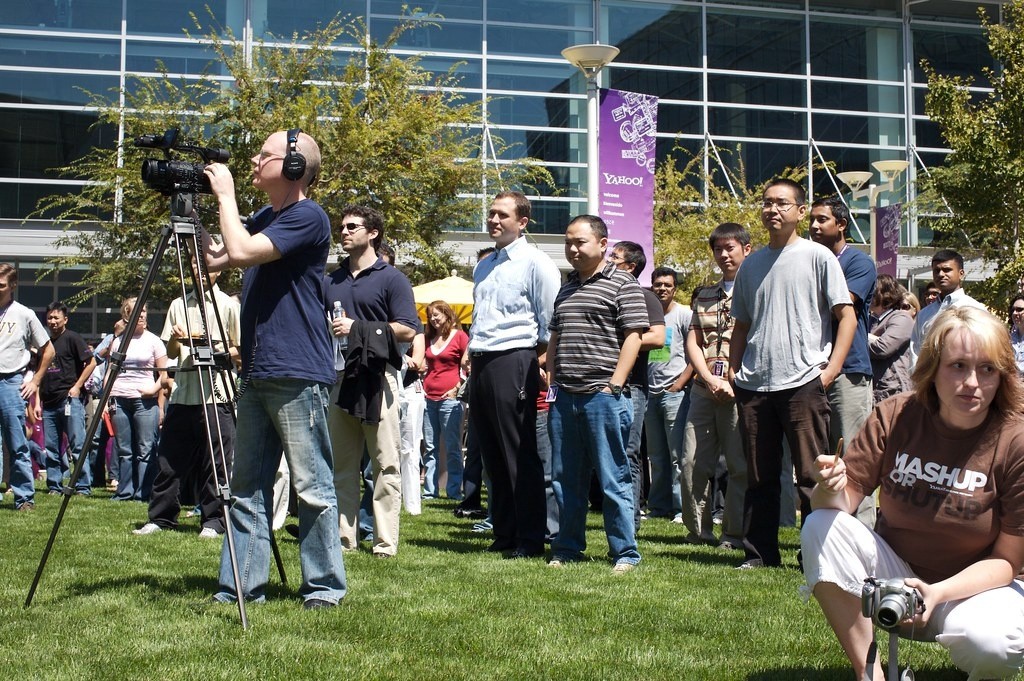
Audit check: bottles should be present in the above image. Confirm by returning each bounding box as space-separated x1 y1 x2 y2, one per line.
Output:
332 301 348 350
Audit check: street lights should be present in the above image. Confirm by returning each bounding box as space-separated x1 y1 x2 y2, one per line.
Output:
559 42 621 217
836 159 911 269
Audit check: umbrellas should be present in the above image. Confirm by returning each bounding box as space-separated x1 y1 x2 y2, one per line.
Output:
410 271 475 325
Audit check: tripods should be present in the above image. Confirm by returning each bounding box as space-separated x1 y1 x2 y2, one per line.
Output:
23 188 290 631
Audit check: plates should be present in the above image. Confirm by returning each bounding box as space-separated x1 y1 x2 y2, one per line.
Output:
176 338 220 347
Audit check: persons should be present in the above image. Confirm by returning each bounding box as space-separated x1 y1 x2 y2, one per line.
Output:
4 301 176 503
466 191 561 557
868 250 986 406
323 206 436 555
424 299 469 500
801 306 1024 680
729 178 857 569
546 215 650 570
462 250 562 540
808 199 877 532
189 127 346 611
0 263 56 510
131 248 293 537
1010 296 1024 373
608 239 728 524
682 222 751 550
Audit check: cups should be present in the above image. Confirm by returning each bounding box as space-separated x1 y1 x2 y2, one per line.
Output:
199 334 212 340
38 470 47 481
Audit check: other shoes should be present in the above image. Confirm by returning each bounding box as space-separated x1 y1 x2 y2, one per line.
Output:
737 559 764 568
131 522 161 534
199 527 219 538
305 599 334 610
614 564 633 573
717 542 733 549
549 554 568 567
19 502 33 510
48 490 58 495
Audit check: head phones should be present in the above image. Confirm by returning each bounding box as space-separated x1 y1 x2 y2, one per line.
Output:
282 127 306 181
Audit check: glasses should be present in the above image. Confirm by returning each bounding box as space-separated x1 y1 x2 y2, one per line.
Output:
761 201 801 210
339 223 366 232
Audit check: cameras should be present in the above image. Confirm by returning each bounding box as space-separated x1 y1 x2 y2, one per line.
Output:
861 577 924 630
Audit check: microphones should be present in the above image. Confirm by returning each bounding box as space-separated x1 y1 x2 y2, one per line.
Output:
133 136 160 148
